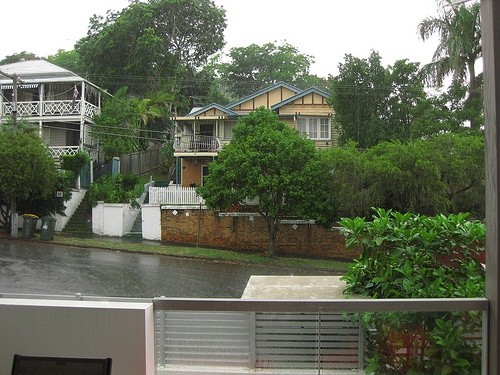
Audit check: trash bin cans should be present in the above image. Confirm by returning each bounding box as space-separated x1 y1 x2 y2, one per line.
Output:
39 217 56 242
20 213 39 239
